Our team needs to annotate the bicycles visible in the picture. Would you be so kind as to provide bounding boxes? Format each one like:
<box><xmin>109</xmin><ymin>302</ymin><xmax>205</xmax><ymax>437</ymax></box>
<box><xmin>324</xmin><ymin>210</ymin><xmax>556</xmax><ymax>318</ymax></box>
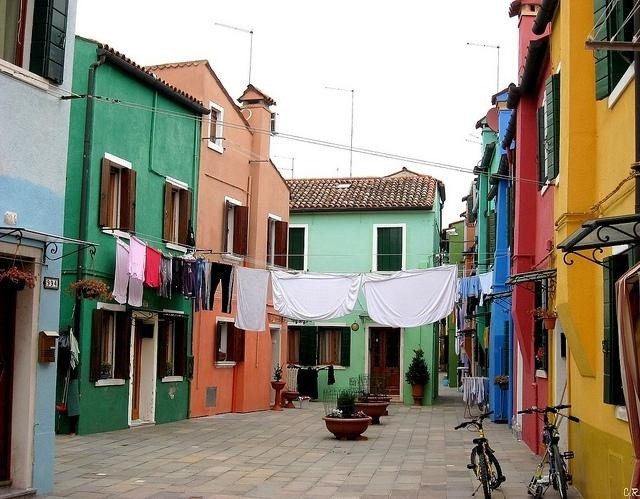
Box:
<box><xmin>454</xmin><ymin>410</ymin><xmax>506</xmax><ymax>499</ymax></box>
<box><xmin>517</xmin><ymin>403</ymin><xmax>579</xmax><ymax>498</ymax></box>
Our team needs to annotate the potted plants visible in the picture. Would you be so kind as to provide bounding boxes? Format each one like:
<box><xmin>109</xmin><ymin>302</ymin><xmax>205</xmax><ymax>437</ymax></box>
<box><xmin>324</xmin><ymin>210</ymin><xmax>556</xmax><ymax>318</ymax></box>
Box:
<box><xmin>493</xmin><ymin>373</ymin><xmax>509</xmax><ymax>391</ymax></box>
<box><xmin>271</xmin><ymin>361</ymin><xmax>286</xmax><ymax>410</ymax></box>
<box><xmin>98</xmin><ymin>360</ymin><xmax>113</xmax><ymax>379</ymax></box>
<box><xmin>322</xmin><ymin>374</ymin><xmax>392</xmax><ymax>440</ymax></box>
<box><xmin>283</xmin><ymin>390</ymin><xmax>299</xmax><ymax>408</ymax></box>
<box><xmin>404</xmin><ymin>347</ymin><xmax>430</xmax><ymax>406</ymax></box>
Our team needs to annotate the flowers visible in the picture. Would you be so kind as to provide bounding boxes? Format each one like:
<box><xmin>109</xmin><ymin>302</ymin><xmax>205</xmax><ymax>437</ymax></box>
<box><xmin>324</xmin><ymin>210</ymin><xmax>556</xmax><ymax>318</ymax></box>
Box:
<box><xmin>530</xmin><ymin>304</ymin><xmax>559</xmax><ymax>319</ymax></box>
<box><xmin>63</xmin><ymin>278</ymin><xmax>115</xmax><ymax>302</ymax></box>
<box><xmin>0</xmin><ymin>265</ymin><xmax>37</xmax><ymax>290</ymax></box>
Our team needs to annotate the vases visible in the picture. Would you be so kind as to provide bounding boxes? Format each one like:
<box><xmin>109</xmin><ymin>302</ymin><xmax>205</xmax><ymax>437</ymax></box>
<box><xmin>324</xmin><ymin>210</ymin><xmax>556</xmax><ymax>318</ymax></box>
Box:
<box><xmin>543</xmin><ymin>318</ymin><xmax>557</xmax><ymax>329</ymax></box>
<box><xmin>4</xmin><ymin>277</ymin><xmax>25</xmax><ymax>292</ymax></box>
<box><xmin>78</xmin><ymin>288</ymin><xmax>97</xmax><ymax>300</ymax></box>
